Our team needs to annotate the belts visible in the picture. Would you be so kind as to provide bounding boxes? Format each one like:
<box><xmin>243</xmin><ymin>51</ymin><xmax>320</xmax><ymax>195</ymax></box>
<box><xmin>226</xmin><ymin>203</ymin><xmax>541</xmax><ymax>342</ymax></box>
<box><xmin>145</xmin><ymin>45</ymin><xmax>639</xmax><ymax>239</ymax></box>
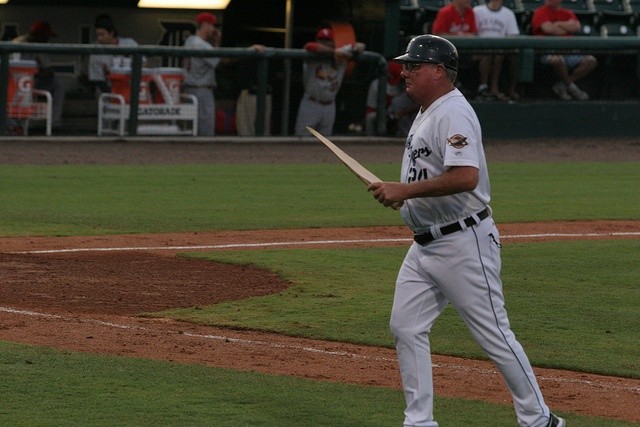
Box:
<box><xmin>308</xmin><ymin>96</ymin><xmax>333</xmax><ymax>105</ymax></box>
<box><xmin>415</xmin><ymin>207</ymin><xmax>488</xmax><ymax>243</ymax></box>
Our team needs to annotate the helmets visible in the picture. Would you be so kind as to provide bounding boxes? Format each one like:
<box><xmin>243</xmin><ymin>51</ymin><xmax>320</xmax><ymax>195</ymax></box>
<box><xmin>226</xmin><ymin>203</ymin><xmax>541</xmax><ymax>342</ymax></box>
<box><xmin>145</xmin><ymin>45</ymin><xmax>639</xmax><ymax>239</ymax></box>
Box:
<box><xmin>316</xmin><ymin>27</ymin><xmax>335</xmax><ymax>42</ymax></box>
<box><xmin>395</xmin><ymin>34</ymin><xmax>459</xmax><ymax>71</ymax></box>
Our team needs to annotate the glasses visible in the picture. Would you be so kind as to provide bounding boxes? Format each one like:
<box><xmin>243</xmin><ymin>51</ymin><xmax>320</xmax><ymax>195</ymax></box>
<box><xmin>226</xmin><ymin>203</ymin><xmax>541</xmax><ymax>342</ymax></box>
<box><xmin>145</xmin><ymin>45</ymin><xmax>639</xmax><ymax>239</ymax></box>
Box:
<box><xmin>404</xmin><ymin>62</ymin><xmax>433</xmax><ymax>68</ymax></box>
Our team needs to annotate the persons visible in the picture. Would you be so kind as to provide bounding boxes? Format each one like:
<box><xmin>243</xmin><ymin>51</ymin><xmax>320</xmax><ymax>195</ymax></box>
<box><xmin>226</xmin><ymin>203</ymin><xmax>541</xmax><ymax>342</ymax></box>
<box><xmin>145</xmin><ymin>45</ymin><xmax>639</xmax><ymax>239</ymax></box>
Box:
<box><xmin>293</xmin><ymin>28</ymin><xmax>365</xmax><ymax>136</ymax></box>
<box><xmin>473</xmin><ymin>0</ymin><xmax>521</xmax><ymax>101</ymax></box>
<box><xmin>8</xmin><ymin>18</ymin><xmax>54</xmax><ymax>91</ymax></box>
<box><xmin>364</xmin><ymin>60</ymin><xmax>419</xmax><ymax>136</ymax></box>
<box><xmin>531</xmin><ymin>0</ymin><xmax>597</xmax><ymax>101</ymax></box>
<box><xmin>431</xmin><ymin>0</ymin><xmax>496</xmax><ymax>101</ymax></box>
<box><xmin>87</xmin><ymin>16</ymin><xmax>148</xmax><ymax>93</ymax></box>
<box><xmin>366</xmin><ymin>33</ymin><xmax>567</xmax><ymax>427</ymax></box>
<box><xmin>179</xmin><ymin>12</ymin><xmax>267</xmax><ymax>136</ymax></box>
<box><xmin>236</xmin><ymin>42</ymin><xmax>273</xmax><ymax>139</ymax></box>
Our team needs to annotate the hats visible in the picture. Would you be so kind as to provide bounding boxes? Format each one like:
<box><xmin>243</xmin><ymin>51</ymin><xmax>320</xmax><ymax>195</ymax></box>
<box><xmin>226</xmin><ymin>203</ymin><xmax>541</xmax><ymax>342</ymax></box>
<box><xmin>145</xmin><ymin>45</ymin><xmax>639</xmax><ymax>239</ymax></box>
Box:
<box><xmin>30</xmin><ymin>20</ymin><xmax>58</xmax><ymax>38</ymax></box>
<box><xmin>94</xmin><ymin>16</ymin><xmax>120</xmax><ymax>34</ymax></box>
<box><xmin>195</xmin><ymin>12</ymin><xmax>218</xmax><ymax>27</ymax></box>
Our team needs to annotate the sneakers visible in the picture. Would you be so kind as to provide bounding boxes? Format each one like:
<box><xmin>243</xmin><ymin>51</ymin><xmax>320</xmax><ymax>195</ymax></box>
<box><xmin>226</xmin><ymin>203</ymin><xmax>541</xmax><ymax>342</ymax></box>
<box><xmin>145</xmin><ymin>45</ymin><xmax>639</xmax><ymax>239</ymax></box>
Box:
<box><xmin>545</xmin><ymin>410</ymin><xmax>565</xmax><ymax>427</ymax></box>
<box><xmin>553</xmin><ymin>81</ymin><xmax>572</xmax><ymax>100</ymax></box>
<box><xmin>567</xmin><ymin>83</ymin><xmax>589</xmax><ymax>101</ymax></box>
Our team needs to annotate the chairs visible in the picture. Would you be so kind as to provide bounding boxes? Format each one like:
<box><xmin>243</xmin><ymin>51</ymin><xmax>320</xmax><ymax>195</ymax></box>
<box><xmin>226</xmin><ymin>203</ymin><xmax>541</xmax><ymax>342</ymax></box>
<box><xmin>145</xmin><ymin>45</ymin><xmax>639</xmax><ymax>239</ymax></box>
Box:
<box><xmin>397</xmin><ymin>0</ymin><xmax>638</xmax><ymax>99</ymax></box>
<box><xmin>156</xmin><ymin>19</ymin><xmax>196</xmax><ymax>68</ymax></box>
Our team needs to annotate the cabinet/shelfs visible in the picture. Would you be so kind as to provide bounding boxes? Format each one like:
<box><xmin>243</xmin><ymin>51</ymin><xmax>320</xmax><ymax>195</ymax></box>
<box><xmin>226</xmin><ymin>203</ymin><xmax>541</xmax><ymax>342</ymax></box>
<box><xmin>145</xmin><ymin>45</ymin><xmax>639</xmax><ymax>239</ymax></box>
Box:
<box><xmin>6</xmin><ymin>88</ymin><xmax>56</xmax><ymax>137</ymax></box>
<box><xmin>96</xmin><ymin>93</ymin><xmax>200</xmax><ymax>137</ymax></box>
